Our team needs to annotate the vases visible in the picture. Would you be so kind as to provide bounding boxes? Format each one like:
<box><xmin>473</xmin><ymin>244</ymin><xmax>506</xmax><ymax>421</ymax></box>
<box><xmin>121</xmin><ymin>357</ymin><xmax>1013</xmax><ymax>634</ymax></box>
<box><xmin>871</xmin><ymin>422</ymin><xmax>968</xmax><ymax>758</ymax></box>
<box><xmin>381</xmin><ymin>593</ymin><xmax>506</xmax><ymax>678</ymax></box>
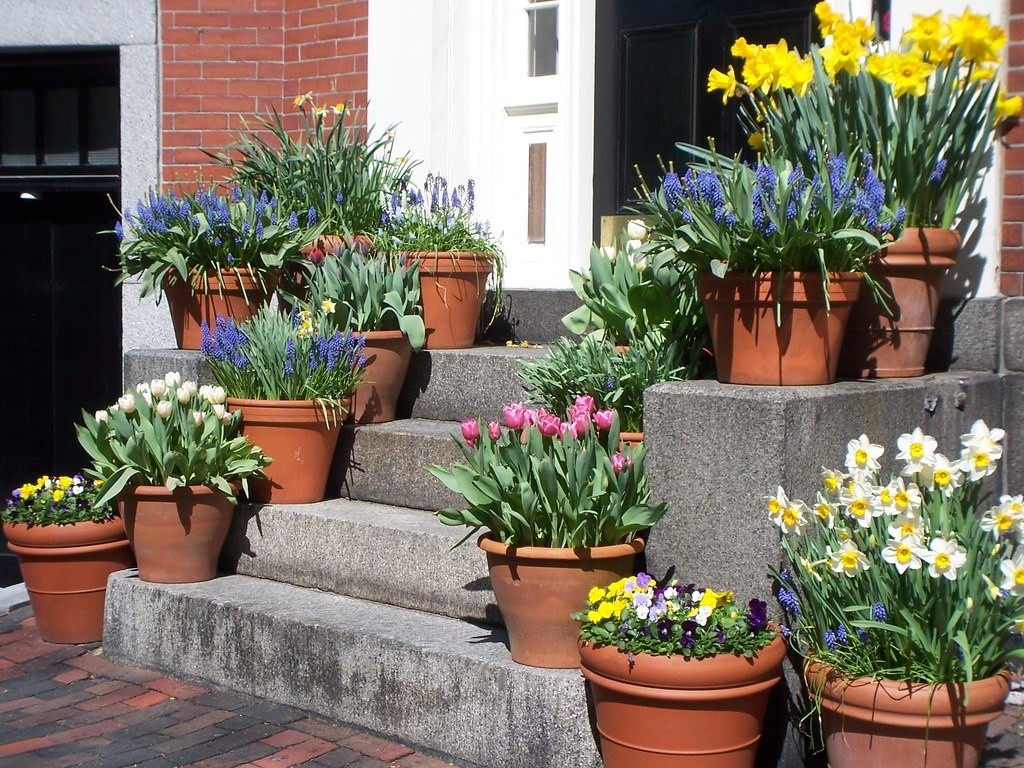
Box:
<box><xmin>285</xmin><ymin>235</ymin><xmax>372</xmax><ymax>310</ymax></box>
<box><xmin>160</xmin><ymin>268</ymin><xmax>283</xmax><ymax>351</ymax></box>
<box><xmin>837</xmin><ymin>226</ymin><xmax>963</xmax><ymax>378</ymax></box>
<box><xmin>576</xmin><ymin>621</ymin><xmax>790</xmax><ymax>768</ymax></box>
<box><xmin>596</xmin><ymin>432</ymin><xmax>643</xmax><ymax>454</ymax></box>
<box><xmin>383</xmin><ymin>250</ymin><xmax>493</xmax><ymax>349</ymax></box>
<box><xmin>3</xmin><ymin>515</ymin><xmax>136</xmax><ymax>644</ymax></box>
<box><xmin>801</xmin><ymin>648</ymin><xmax>1012</xmax><ymax>767</ymax></box>
<box><xmin>338</xmin><ymin>329</ymin><xmax>412</xmax><ymax>424</ymax></box>
<box><xmin>477</xmin><ymin>531</ymin><xmax>646</xmax><ymax>668</ymax></box>
<box><xmin>696</xmin><ymin>271</ymin><xmax>864</xmax><ymax>386</ymax></box>
<box><xmin>224</xmin><ymin>397</ymin><xmax>352</xmax><ymax>504</ymax></box>
<box><xmin>614</xmin><ymin>347</ymin><xmax>712</xmax><ymax>378</ymax></box>
<box><xmin>114</xmin><ymin>481</ymin><xmax>245</xmax><ymax>584</ymax></box>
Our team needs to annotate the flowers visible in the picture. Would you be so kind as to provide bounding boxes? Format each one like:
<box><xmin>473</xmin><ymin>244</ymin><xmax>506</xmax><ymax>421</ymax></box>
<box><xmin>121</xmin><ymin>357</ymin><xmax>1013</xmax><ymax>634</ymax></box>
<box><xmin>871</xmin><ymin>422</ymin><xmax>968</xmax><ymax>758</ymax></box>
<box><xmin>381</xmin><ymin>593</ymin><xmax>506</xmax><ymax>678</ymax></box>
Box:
<box><xmin>515</xmin><ymin>0</ymin><xmax>1024</xmax><ymax>432</ymax></box>
<box><xmin>569</xmin><ymin>565</ymin><xmax>786</xmax><ymax>668</ymax></box>
<box><xmin>73</xmin><ymin>371</ymin><xmax>275</xmax><ymax>506</ymax></box>
<box><xmin>761</xmin><ymin>417</ymin><xmax>1024</xmax><ymax>768</ymax></box>
<box><xmin>92</xmin><ymin>90</ymin><xmax>509</xmax><ymax>431</ymax></box>
<box><xmin>0</xmin><ymin>473</ymin><xmax>119</xmax><ymax>527</ymax></box>
<box><xmin>421</xmin><ymin>393</ymin><xmax>670</xmax><ymax>554</ymax></box>
